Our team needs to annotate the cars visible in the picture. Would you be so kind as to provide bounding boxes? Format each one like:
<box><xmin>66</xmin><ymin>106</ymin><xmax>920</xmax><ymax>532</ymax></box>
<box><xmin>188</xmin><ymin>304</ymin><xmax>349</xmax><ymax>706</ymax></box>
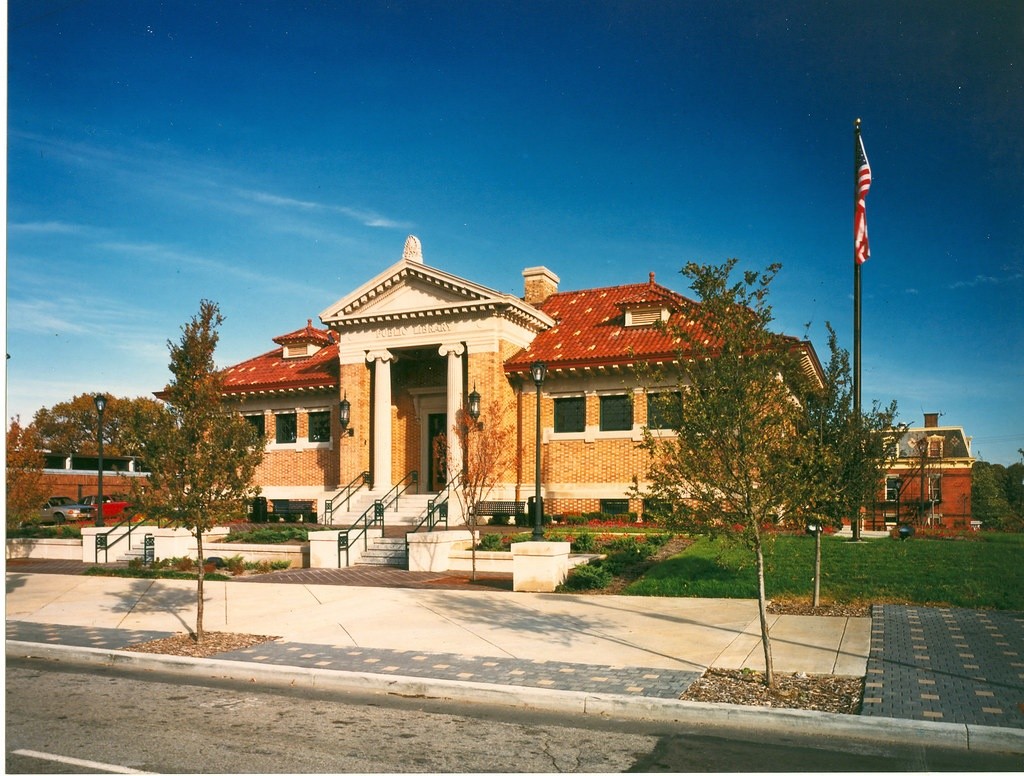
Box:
<box><xmin>78</xmin><ymin>495</ymin><xmax>133</xmax><ymax>519</ymax></box>
<box><xmin>29</xmin><ymin>494</ymin><xmax>96</xmax><ymax>524</ymax></box>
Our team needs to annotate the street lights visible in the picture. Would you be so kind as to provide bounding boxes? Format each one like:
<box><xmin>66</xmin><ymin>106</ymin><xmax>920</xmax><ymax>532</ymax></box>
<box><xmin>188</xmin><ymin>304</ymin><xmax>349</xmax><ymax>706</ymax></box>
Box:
<box><xmin>92</xmin><ymin>393</ymin><xmax>108</xmax><ymax>528</ymax></box>
<box><xmin>530</xmin><ymin>357</ymin><xmax>548</xmax><ymax>542</ymax></box>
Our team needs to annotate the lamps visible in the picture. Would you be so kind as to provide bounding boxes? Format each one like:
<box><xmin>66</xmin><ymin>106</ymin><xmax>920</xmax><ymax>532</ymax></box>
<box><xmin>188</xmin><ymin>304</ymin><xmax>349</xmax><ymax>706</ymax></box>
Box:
<box><xmin>468</xmin><ymin>379</ymin><xmax>483</xmax><ymax>431</ymax></box>
<box><xmin>339</xmin><ymin>390</ymin><xmax>354</xmax><ymax>436</ymax></box>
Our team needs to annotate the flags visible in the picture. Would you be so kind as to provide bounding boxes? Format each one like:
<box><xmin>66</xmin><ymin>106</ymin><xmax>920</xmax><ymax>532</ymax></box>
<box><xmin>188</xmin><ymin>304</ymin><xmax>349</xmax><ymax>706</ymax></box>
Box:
<box><xmin>854</xmin><ymin>128</ymin><xmax>872</xmax><ymax>265</ymax></box>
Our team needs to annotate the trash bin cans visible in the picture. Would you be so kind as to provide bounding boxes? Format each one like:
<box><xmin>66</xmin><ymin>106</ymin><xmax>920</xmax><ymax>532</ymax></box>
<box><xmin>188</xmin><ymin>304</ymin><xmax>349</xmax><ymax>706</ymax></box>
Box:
<box><xmin>252</xmin><ymin>496</ymin><xmax>268</xmax><ymax>523</ymax></box>
<box><xmin>527</xmin><ymin>496</ymin><xmax>544</xmax><ymax>527</ymax></box>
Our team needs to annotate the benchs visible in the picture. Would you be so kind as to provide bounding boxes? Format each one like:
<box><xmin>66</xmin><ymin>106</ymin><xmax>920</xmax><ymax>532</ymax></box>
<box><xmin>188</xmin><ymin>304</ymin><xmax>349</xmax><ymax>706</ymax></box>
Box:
<box><xmin>468</xmin><ymin>501</ymin><xmax>526</xmax><ymax>525</ymax></box>
<box><xmin>269</xmin><ymin>499</ymin><xmax>315</xmax><ymax>524</ymax></box>
